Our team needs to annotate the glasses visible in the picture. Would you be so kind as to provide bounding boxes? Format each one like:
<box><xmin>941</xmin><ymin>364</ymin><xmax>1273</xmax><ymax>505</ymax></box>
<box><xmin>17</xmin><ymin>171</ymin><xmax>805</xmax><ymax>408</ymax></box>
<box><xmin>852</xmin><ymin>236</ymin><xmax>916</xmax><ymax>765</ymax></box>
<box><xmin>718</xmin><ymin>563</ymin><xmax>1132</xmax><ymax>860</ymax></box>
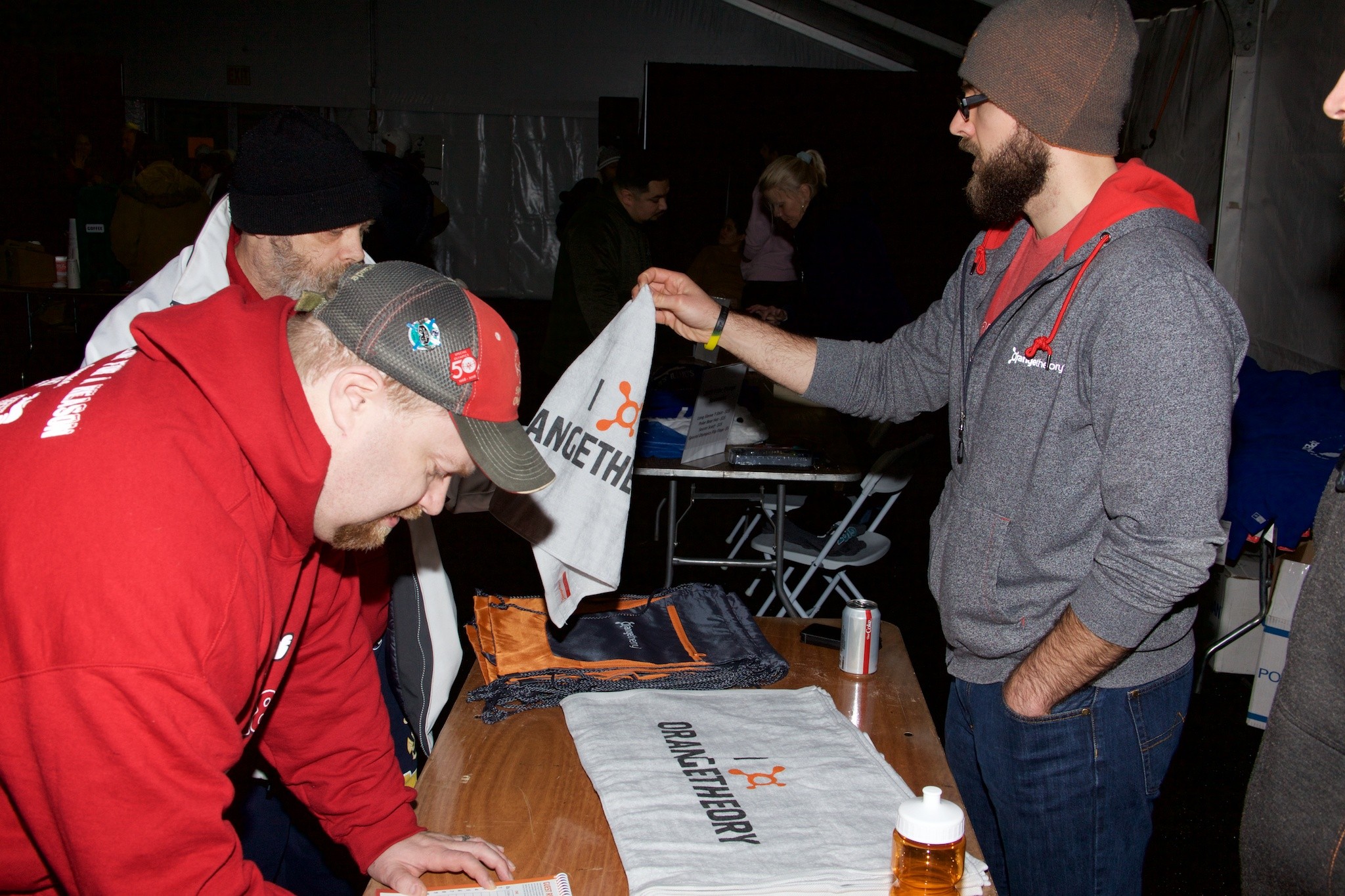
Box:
<box><xmin>956</xmin><ymin>94</ymin><xmax>989</xmax><ymax>122</ymax></box>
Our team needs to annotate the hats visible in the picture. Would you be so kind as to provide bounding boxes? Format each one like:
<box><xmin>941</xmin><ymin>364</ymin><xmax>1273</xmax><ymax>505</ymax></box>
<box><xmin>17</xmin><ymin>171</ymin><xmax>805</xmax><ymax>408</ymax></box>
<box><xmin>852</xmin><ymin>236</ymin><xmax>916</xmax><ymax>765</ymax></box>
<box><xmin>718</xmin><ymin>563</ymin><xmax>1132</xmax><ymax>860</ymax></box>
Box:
<box><xmin>957</xmin><ymin>0</ymin><xmax>1139</xmax><ymax>158</ymax></box>
<box><xmin>293</xmin><ymin>258</ymin><xmax>556</xmax><ymax>495</ymax></box>
<box><xmin>230</xmin><ymin>115</ymin><xmax>392</xmax><ymax>236</ymax></box>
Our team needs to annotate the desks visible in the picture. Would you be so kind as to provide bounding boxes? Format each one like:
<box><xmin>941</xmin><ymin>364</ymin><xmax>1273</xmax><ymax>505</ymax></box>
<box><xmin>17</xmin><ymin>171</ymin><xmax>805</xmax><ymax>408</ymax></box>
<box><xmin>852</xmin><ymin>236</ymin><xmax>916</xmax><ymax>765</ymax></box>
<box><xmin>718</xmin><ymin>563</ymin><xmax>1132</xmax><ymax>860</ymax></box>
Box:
<box><xmin>1192</xmin><ymin>357</ymin><xmax>1345</xmax><ymax>694</ymax></box>
<box><xmin>630</xmin><ymin>370</ymin><xmax>862</xmax><ymax>621</ymax></box>
<box><xmin>360</xmin><ymin>615</ymin><xmax>997</xmax><ymax>896</ymax></box>
<box><xmin>0</xmin><ymin>281</ymin><xmax>134</xmax><ymax>390</ymax></box>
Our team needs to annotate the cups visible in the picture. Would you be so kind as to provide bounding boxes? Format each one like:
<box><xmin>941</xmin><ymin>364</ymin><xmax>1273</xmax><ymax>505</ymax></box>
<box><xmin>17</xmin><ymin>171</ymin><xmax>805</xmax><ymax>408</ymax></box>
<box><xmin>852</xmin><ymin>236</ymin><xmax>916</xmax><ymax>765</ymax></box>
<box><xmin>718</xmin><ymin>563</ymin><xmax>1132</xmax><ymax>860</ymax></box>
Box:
<box><xmin>55</xmin><ymin>256</ymin><xmax>67</xmax><ymax>276</ymax></box>
<box><xmin>890</xmin><ymin>786</ymin><xmax>968</xmax><ymax>896</ymax></box>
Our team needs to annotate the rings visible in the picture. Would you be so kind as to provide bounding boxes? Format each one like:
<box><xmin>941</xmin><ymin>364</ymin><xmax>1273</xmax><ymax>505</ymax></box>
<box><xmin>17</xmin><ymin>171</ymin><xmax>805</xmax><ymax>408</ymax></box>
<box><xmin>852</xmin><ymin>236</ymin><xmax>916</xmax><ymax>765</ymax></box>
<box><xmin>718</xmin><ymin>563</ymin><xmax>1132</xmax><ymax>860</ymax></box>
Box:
<box><xmin>461</xmin><ymin>835</ymin><xmax>471</xmax><ymax>841</ymax></box>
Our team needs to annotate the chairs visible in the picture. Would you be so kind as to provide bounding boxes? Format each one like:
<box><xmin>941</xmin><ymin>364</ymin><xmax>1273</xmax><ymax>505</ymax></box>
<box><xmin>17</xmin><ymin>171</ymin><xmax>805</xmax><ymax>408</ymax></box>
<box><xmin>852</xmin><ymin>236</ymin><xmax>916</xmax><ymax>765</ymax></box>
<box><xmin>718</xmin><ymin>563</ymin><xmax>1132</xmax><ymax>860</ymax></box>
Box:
<box><xmin>721</xmin><ymin>490</ymin><xmax>806</xmax><ymax>598</ymax></box>
<box><xmin>746</xmin><ymin>430</ymin><xmax>937</xmax><ymax>620</ymax></box>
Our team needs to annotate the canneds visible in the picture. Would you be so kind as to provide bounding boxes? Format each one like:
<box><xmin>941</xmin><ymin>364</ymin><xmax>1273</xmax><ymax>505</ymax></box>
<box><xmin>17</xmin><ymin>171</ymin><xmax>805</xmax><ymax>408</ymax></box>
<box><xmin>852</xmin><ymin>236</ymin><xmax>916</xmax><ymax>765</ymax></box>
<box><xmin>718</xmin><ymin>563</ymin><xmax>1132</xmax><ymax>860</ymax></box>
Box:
<box><xmin>839</xmin><ymin>599</ymin><xmax>881</xmax><ymax>674</ymax></box>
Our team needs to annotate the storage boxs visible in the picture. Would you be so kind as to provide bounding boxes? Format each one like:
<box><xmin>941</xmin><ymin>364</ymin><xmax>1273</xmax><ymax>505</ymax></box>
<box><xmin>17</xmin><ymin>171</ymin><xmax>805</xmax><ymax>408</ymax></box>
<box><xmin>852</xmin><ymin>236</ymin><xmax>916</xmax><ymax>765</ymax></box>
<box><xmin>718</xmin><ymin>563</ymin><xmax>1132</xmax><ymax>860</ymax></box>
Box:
<box><xmin>1203</xmin><ymin>557</ymin><xmax>1276</xmax><ymax>677</ymax></box>
<box><xmin>0</xmin><ymin>240</ymin><xmax>58</xmax><ymax>289</ymax></box>
<box><xmin>1246</xmin><ymin>540</ymin><xmax>1316</xmax><ymax>731</ymax></box>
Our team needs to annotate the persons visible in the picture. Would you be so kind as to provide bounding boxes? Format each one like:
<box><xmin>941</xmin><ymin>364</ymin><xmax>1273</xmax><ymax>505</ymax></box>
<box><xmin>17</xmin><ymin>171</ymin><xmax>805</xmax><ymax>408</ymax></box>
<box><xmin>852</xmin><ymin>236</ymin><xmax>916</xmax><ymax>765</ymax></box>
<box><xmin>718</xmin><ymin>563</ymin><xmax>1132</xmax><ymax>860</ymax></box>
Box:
<box><xmin>9</xmin><ymin>110</ymin><xmax>480</xmax><ymax>345</ymax></box>
<box><xmin>689</xmin><ymin>215</ymin><xmax>751</xmax><ymax>314</ymax></box>
<box><xmin>739</xmin><ymin>124</ymin><xmax>805</xmax><ymax>336</ymax></box>
<box><xmin>579</xmin><ymin>146</ymin><xmax>626</xmax><ymax>217</ymax></box>
<box><xmin>1234</xmin><ymin>59</ymin><xmax>1345</xmax><ymax>896</ymax></box>
<box><xmin>626</xmin><ymin>0</ymin><xmax>1251</xmax><ymax>894</ymax></box>
<box><xmin>2</xmin><ymin>259</ymin><xmax>556</xmax><ymax>895</ymax></box>
<box><xmin>80</xmin><ymin>105</ymin><xmax>466</xmax><ymax>896</ymax></box>
<box><xmin>541</xmin><ymin>148</ymin><xmax>673</xmax><ymax>601</ymax></box>
<box><xmin>747</xmin><ymin>149</ymin><xmax>920</xmax><ymax>504</ymax></box>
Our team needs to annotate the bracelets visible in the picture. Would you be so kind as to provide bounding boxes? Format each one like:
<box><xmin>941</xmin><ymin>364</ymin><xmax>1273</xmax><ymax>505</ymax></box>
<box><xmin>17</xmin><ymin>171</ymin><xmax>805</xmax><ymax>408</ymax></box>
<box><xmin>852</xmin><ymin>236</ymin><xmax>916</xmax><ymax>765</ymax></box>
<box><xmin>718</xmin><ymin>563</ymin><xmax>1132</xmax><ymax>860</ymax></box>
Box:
<box><xmin>705</xmin><ymin>305</ymin><xmax>730</xmax><ymax>350</ymax></box>
